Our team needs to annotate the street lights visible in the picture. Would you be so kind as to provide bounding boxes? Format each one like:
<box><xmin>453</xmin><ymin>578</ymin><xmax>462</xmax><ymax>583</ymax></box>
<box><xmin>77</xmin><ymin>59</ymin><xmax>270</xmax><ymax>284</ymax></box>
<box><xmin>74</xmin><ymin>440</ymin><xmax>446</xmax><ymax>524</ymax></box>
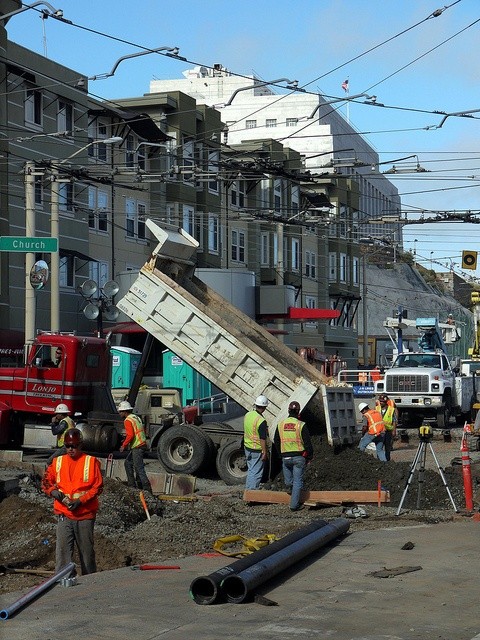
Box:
<box><xmin>47</xmin><ymin>136</ymin><xmax>124</xmax><ymax>333</ymax></box>
<box><xmin>276</xmin><ymin>207</ymin><xmax>329</xmax><ymax>344</ymax></box>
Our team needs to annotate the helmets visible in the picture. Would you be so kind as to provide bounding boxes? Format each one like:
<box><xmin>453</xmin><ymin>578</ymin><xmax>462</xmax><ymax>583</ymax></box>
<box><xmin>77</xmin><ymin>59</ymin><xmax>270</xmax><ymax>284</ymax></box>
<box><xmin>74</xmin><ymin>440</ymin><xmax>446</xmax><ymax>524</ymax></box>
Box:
<box><xmin>254</xmin><ymin>395</ymin><xmax>268</xmax><ymax>407</ymax></box>
<box><xmin>117</xmin><ymin>401</ymin><xmax>133</xmax><ymax>411</ymax></box>
<box><xmin>289</xmin><ymin>401</ymin><xmax>300</xmax><ymax>410</ymax></box>
<box><xmin>379</xmin><ymin>394</ymin><xmax>388</xmax><ymax>401</ymax></box>
<box><xmin>55</xmin><ymin>403</ymin><xmax>70</xmax><ymax>413</ymax></box>
<box><xmin>358</xmin><ymin>403</ymin><xmax>368</xmax><ymax>412</ymax></box>
<box><xmin>64</xmin><ymin>428</ymin><xmax>82</xmax><ymax>442</ymax></box>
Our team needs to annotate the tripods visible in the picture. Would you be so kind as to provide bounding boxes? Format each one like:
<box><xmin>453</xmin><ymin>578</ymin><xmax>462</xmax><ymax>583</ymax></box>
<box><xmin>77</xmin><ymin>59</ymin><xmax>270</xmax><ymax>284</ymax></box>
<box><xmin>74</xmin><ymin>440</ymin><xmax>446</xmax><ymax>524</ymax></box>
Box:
<box><xmin>397</xmin><ymin>437</ymin><xmax>461</xmax><ymax>516</ymax></box>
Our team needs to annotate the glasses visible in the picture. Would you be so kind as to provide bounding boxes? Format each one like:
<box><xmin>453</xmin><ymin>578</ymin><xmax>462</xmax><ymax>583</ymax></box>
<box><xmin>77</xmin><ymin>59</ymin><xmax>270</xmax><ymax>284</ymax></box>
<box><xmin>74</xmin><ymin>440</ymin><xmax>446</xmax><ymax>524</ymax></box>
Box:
<box><xmin>65</xmin><ymin>443</ymin><xmax>78</xmax><ymax>449</ymax></box>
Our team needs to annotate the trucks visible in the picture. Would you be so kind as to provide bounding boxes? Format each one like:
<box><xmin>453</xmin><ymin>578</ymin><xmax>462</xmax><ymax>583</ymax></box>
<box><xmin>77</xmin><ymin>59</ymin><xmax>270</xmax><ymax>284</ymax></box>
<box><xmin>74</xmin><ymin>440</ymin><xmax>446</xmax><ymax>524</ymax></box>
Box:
<box><xmin>0</xmin><ymin>218</ymin><xmax>360</xmax><ymax>487</ymax></box>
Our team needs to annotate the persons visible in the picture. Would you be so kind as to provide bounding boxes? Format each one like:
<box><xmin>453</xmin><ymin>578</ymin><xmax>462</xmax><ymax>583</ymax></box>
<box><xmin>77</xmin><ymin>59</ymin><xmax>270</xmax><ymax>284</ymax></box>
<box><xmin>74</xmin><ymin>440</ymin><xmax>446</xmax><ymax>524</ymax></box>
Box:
<box><xmin>375</xmin><ymin>399</ymin><xmax>399</xmax><ymax>420</ymax></box>
<box><xmin>274</xmin><ymin>401</ymin><xmax>313</xmax><ymax>511</ymax></box>
<box><xmin>40</xmin><ymin>428</ymin><xmax>103</xmax><ymax>575</ymax></box>
<box><xmin>118</xmin><ymin>401</ymin><xmax>152</xmax><ymax>494</ymax></box>
<box><xmin>358</xmin><ymin>403</ymin><xmax>387</xmax><ymax>461</ymax></box>
<box><xmin>55</xmin><ymin>348</ymin><xmax>62</xmax><ymax>368</ymax></box>
<box><xmin>243</xmin><ymin>395</ymin><xmax>269</xmax><ymax>490</ymax></box>
<box><xmin>375</xmin><ymin>394</ymin><xmax>396</xmax><ymax>461</ymax></box>
<box><xmin>46</xmin><ymin>404</ymin><xmax>77</xmax><ymax>466</ymax></box>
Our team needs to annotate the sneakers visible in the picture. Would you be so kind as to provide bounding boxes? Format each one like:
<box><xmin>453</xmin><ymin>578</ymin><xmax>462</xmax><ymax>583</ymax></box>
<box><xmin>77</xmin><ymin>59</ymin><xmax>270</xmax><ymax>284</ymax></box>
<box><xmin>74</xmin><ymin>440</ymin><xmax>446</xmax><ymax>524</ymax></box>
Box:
<box><xmin>292</xmin><ymin>503</ymin><xmax>304</xmax><ymax>510</ymax></box>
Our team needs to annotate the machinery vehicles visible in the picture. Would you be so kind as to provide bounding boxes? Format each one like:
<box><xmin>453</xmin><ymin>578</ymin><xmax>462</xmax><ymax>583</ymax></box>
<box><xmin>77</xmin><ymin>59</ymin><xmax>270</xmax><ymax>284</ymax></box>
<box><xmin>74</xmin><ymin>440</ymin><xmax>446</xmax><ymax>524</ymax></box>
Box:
<box><xmin>374</xmin><ymin>317</ymin><xmax>480</xmax><ymax>428</ymax></box>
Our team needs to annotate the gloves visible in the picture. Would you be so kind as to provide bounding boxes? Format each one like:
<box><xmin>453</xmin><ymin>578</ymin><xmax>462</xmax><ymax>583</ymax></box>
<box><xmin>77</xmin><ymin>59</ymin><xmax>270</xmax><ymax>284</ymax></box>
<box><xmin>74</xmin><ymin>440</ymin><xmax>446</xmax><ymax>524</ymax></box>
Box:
<box><xmin>68</xmin><ymin>499</ymin><xmax>80</xmax><ymax>510</ymax></box>
<box><xmin>120</xmin><ymin>446</ymin><xmax>124</xmax><ymax>452</ymax></box>
<box><xmin>51</xmin><ymin>490</ymin><xmax>64</xmax><ymax>501</ymax></box>
<box><xmin>52</xmin><ymin>417</ymin><xmax>57</xmax><ymax>422</ymax></box>
